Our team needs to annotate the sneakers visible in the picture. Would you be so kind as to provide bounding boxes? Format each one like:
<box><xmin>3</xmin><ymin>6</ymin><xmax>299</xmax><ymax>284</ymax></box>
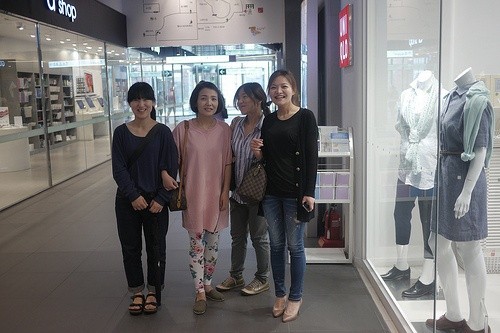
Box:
<box><xmin>240</xmin><ymin>277</ymin><xmax>270</xmax><ymax>295</ymax></box>
<box><xmin>215</xmin><ymin>276</ymin><xmax>246</xmax><ymax>292</ymax></box>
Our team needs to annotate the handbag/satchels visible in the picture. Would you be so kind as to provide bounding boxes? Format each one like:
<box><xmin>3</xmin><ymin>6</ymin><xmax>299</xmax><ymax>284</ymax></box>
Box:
<box><xmin>237</xmin><ymin>152</ymin><xmax>270</xmax><ymax>206</ymax></box>
<box><xmin>167</xmin><ymin>119</ymin><xmax>189</xmax><ymax>212</ymax></box>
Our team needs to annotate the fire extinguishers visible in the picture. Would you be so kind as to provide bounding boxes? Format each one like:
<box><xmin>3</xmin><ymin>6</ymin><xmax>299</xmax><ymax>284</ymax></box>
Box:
<box><xmin>322</xmin><ymin>204</ymin><xmax>340</xmax><ymax>240</ymax></box>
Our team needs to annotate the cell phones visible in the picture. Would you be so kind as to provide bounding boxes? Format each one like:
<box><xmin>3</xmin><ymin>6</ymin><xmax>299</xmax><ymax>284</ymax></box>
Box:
<box><xmin>302</xmin><ymin>203</ymin><xmax>310</xmax><ymax>213</ymax></box>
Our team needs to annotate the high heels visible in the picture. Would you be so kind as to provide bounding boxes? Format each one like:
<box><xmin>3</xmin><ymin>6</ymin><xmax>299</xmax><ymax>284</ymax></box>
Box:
<box><xmin>271</xmin><ymin>295</ymin><xmax>287</xmax><ymax>317</ymax></box>
<box><xmin>282</xmin><ymin>298</ymin><xmax>303</xmax><ymax>322</ymax></box>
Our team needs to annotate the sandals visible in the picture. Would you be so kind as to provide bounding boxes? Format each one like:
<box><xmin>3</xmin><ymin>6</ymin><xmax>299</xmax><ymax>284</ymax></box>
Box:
<box><xmin>128</xmin><ymin>294</ymin><xmax>146</xmax><ymax>315</ymax></box>
<box><xmin>142</xmin><ymin>293</ymin><xmax>158</xmax><ymax>314</ymax></box>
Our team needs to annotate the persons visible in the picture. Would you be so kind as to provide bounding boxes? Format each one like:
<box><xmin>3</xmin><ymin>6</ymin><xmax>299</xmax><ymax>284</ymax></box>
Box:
<box><xmin>161</xmin><ymin>80</ymin><xmax>232</xmax><ymax>314</ymax></box>
<box><xmin>426</xmin><ymin>68</ymin><xmax>495</xmax><ymax>333</ymax></box>
<box><xmin>157</xmin><ymin>86</ymin><xmax>179</xmax><ymax>123</ymax></box>
<box><xmin>216</xmin><ymin>96</ymin><xmax>226</xmax><ymax>122</ymax></box>
<box><xmin>250</xmin><ymin>70</ymin><xmax>318</xmax><ymax>323</ymax></box>
<box><xmin>381</xmin><ymin>71</ymin><xmax>449</xmax><ymax>298</ymax></box>
<box><xmin>215</xmin><ymin>82</ymin><xmax>272</xmax><ymax>295</ymax></box>
<box><xmin>112</xmin><ymin>82</ymin><xmax>179</xmax><ymax>313</ymax></box>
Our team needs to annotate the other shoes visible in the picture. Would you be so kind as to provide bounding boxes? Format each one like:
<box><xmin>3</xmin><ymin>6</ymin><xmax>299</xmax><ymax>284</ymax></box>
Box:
<box><xmin>426</xmin><ymin>313</ymin><xmax>466</xmax><ymax>330</ymax></box>
<box><xmin>192</xmin><ymin>297</ymin><xmax>207</xmax><ymax>315</ymax></box>
<box><xmin>455</xmin><ymin>321</ymin><xmax>491</xmax><ymax>333</ymax></box>
<box><xmin>206</xmin><ymin>288</ymin><xmax>225</xmax><ymax>302</ymax></box>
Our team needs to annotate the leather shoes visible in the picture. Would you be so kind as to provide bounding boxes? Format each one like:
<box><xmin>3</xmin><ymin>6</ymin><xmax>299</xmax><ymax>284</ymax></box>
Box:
<box><xmin>380</xmin><ymin>266</ymin><xmax>411</xmax><ymax>281</ymax></box>
<box><xmin>402</xmin><ymin>279</ymin><xmax>435</xmax><ymax>298</ymax></box>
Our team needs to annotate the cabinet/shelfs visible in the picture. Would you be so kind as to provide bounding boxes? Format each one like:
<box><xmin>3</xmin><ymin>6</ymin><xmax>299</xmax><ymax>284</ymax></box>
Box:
<box><xmin>289</xmin><ymin>126</ymin><xmax>354</xmax><ymax>263</ymax></box>
<box><xmin>17</xmin><ymin>71</ymin><xmax>79</xmax><ymax>156</ymax></box>
